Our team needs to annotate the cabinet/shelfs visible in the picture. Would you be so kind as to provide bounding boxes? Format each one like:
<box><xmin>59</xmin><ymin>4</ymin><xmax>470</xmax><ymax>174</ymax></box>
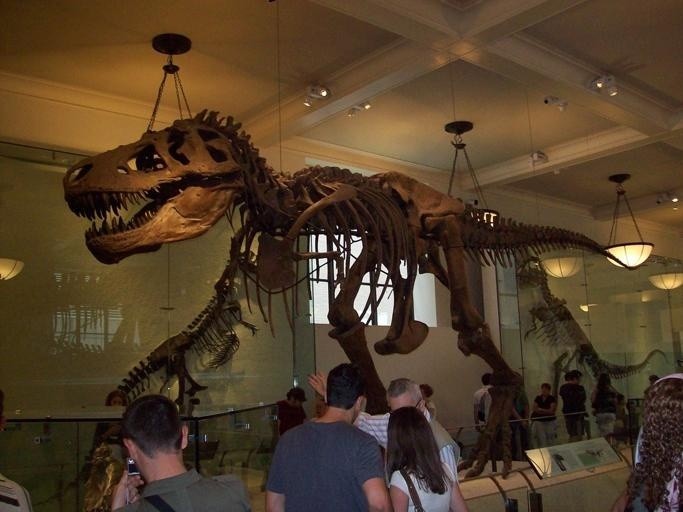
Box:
<box><xmin>0</xmin><ymin>400</ymin><xmax>286</xmax><ymax>512</ymax></box>
<box><xmin>457</xmin><ymin>441</ymin><xmax>638</xmax><ymax>512</ymax></box>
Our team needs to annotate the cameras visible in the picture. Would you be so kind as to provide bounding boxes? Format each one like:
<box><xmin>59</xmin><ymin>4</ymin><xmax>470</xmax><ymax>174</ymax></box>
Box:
<box><xmin>126</xmin><ymin>457</ymin><xmax>139</xmax><ymax>475</ymax></box>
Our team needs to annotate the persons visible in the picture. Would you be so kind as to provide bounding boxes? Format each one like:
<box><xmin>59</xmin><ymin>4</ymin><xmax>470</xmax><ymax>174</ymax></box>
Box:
<box><xmin>473</xmin><ymin>376</ymin><xmax>530</xmax><ymax>461</ymax></box>
<box><xmin>264</xmin><ymin>362</ymin><xmax>394</xmax><ymax>512</ymax></box>
<box><xmin>610</xmin><ymin>373</ymin><xmax>683</xmax><ymax>512</ymax></box>
<box><xmin>309</xmin><ymin>372</ymin><xmax>461</xmax><ymax>476</ymax></box>
<box><xmin>420</xmin><ymin>384</ymin><xmax>436</xmax><ymax>418</ymax></box>
<box><xmin>386</xmin><ymin>406</ymin><xmax>470</xmax><ymax>512</ymax></box>
<box><xmin>533</xmin><ymin>372</ymin><xmax>623</xmax><ymax>447</ymax></box>
<box><xmin>91</xmin><ymin>389</ymin><xmax>128</xmax><ymax>454</ymax></box>
<box><xmin>274</xmin><ymin>387</ymin><xmax>308</xmax><ymax>443</ymax></box>
<box><xmin>104</xmin><ymin>395</ymin><xmax>252</xmax><ymax>511</ymax></box>
<box><xmin>1</xmin><ymin>390</ymin><xmax>33</xmax><ymax>511</ymax></box>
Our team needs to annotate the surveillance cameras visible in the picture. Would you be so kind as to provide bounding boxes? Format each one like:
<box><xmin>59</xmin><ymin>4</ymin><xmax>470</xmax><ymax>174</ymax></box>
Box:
<box><xmin>543</xmin><ymin>95</ymin><xmax>568</xmax><ymax>107</ymax></box>
<box><xmin>656</xmin><ymin>191</ymin><xmax>669</xmax><ymax>204</ymax></box>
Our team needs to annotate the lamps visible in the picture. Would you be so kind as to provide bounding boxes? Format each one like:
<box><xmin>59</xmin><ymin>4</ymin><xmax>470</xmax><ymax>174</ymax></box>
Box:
<box><xmin>586</xmin><ymin>70</ymin><xmax>621</xmax><ymax>99</ymax></box>
<box><xmin>540</xmin><ymin>252</ymin><xmax>586</xmax><ymax>282</ymax></box>
<box><xmin>601</xmin><ymin>172</ymin><xmax>656</xmax><ymax>271</ymax></box>
<box><xmin>665</xmin><ymin>191</ymin><xmax>682</xmax><ymax>214</ymax></box>
<box><xmin>542</xmin><ymin>92</ymin><xmax>570</xmax><ymax>115</ymax></box>
<box><xmin>645</xmin><ymin>262</ymin><xmax>682</xmax><ymax>292</ymax></box>
<box><xmin>441</xmin><ymin>117</ymin><xmax>501</xmax><ymax>230</ymax></box>
<box><xmin>343</xmin><ymin>101</ymin><xmax>373</xmax><ymax>122</ymax></box>
<box><xmin>0</xmin><ymin>254</ymin><xmax>27</xmax><ymax>282</ymax></box>
<box><xmin>298</xmin><ymin>81</ymin><xmax>333</xmax><ymax>111</ymax></box>
